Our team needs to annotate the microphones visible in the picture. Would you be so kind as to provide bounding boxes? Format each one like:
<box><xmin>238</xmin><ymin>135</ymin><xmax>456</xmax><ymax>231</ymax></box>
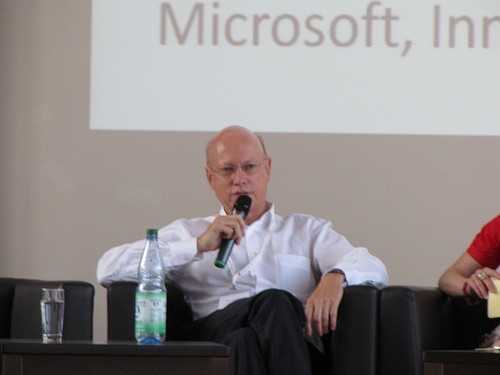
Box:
<box><xmin>215</xmin><ymin>194</ymin><xmax>252</xmax><ymax>268</ymax></box>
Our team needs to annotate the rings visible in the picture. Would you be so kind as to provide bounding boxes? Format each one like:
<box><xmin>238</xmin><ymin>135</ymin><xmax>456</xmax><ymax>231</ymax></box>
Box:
<box><xmin>478</xmin><ymin>271</ymin><xmax>489</xmax><ymax>281</ymax></box>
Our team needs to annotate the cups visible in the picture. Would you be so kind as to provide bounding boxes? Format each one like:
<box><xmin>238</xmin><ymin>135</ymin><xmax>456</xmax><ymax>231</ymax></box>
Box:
<box><xmin>40</xmin><ymin>288</ymin><xmax>64</xmax><ymax>343</ymax></box>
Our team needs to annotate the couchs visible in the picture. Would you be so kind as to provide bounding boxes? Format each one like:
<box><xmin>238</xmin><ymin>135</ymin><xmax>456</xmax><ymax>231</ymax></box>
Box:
<box><xmin>107</xmin><ymin>282</ymin><xmax>500</xmax><ymax>375</ymax></box>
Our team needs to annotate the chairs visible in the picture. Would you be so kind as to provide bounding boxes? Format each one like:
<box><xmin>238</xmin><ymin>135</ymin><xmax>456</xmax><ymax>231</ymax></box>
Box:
<box><xmin>0</xmin><ymin>277</ymin><xmax>95</xmax><ymax>344</ymax></box>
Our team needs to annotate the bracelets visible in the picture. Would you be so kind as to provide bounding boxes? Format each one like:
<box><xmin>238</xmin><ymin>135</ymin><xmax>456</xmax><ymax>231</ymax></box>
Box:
<box><xmin>463</xmin><ymin>281</ymin><xmax>471</xmax><ymax>298</ymax></box>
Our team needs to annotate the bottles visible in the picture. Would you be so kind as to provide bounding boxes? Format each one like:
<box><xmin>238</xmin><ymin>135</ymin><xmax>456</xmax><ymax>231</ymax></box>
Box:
<box><xmin>134</xmin><ymin>228</ymin><xmax>167</xmax><ymax>344</ymax></box>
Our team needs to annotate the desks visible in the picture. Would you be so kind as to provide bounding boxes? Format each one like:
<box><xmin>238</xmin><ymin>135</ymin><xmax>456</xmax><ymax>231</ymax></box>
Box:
<box><xmin>422</xmin><ymin>350</ymin><xmax>500</xmax><ymax>375</ymax></box>
<box><xmin>0</xmin><ymin>340</ymin><xmax>229</xmax><ymax>375</ymax></box>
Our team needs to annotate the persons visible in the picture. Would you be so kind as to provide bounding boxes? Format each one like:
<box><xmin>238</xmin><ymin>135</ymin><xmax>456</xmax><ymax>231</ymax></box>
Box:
<box><xmin>97</xmin><ymin>125</ymin><xmax>388</xmax><ymax>375</ymax></box>
<box><xmin>438</xmin><ymin>214</ymin><xmax>500</xmax><ymax>300</ymax></box>
